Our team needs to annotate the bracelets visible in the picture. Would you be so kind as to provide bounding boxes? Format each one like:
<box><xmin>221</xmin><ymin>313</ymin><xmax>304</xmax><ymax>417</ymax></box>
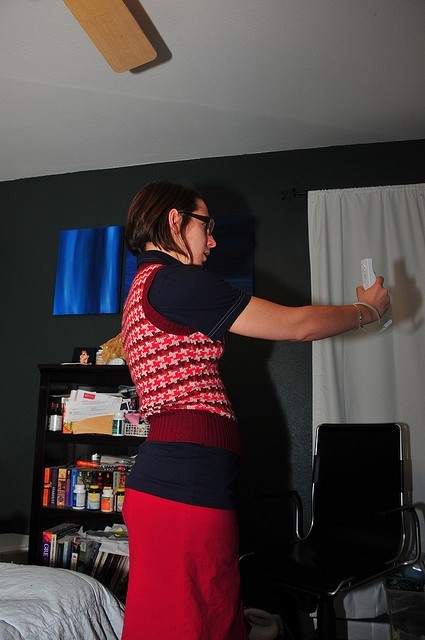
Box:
<box><xmin>352</xmin><ymin>304</ymin><xmax>364</xmax><ymax>332</ymax></box>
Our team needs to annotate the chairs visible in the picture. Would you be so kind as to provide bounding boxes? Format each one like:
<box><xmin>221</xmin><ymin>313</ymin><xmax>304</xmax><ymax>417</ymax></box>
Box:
<box><xmin>239</xmin><ymin>422</ymin><xmax>424</xmax><ymax>639</ymax></box>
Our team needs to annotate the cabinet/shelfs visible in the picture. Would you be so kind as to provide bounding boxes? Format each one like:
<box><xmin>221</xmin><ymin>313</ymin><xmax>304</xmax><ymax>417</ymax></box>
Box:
<box><xmin>29</xmin><ymin>362</ymin><xmax>150</xmax><ymax>601</ymax></box>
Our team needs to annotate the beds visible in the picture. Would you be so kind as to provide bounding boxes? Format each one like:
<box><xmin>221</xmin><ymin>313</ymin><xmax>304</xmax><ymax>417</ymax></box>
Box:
<box><xmin>0</xmin><ymin>562</ymin><xmax>125</xmax><ymax>640</ymax></box>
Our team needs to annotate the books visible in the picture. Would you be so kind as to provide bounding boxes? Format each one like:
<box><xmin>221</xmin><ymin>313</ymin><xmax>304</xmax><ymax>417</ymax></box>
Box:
<box><xmin>40</xmin><ymin>465</ymin><xmax>132</xmax><ymax>518</ymax></box>
<box><xmin>35</xmin><ymin>522</ymin><xmax>130</xmax><ymax>605</ymax></box>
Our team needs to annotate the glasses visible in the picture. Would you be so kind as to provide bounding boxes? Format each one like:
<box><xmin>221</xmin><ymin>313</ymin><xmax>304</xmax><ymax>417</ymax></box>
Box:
<box><xmin>179</xmin><ymin>209</ymin><xmax>217</xmax><ymax>236</ymax></box>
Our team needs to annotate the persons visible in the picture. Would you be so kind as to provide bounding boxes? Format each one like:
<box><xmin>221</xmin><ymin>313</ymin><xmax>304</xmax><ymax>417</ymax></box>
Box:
<box><xmin>120</xmin><ymin>179</ymin><xmax>391</xmax><ymax>639</ymax></box>
